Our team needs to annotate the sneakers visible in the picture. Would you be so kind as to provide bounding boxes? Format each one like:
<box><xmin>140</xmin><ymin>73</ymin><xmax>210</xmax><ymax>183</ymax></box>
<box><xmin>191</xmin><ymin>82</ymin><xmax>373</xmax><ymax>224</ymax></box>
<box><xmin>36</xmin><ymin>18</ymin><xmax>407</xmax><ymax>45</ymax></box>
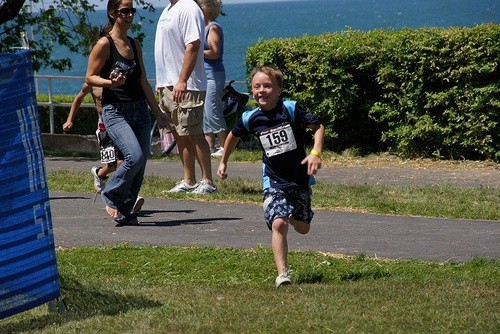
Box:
<box><xmin>130</xmin><ymin>196</ymin><xmax>144</xmax><ymax>213</ymax></box>
<box><xmin>91</xmin><ymin>166</ymin><xmax>109</xmax><ymax>193</ymax></box>
<box><xmin>210</xmin><ymin>146</ymin><xmax>224</xmax><ymax>157</ymax></box>
<box><xmin>186</xmin><ymin>181</ymin><xmax>217</xmax><ymax>194</ymax></box>
<box><xmin>163</xmin><ymin>180</ymin><xmax>200</xmax><ymax>194</ymax></box>
<box><xmin>276</xmin><ymin>267</ymin><xmax>292</xmax><ymax>288</ymax></box>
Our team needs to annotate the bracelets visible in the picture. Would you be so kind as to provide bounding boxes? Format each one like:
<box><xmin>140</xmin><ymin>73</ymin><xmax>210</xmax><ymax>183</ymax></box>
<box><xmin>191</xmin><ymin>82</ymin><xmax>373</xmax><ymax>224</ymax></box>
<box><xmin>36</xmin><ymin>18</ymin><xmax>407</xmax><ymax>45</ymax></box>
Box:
<box><xmin>311</xmin><ymin>151</ymin><xmax>322</xmax><ymax>158</ymax></box>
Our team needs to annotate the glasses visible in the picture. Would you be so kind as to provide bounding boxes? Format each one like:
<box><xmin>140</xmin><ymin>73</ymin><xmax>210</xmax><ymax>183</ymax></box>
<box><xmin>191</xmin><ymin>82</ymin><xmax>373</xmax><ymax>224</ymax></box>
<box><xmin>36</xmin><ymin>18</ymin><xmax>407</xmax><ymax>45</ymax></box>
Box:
<box><xmin>115</xmin><ymin>8</ymin><xmax>136</xmax><ymax>16</ymax></box>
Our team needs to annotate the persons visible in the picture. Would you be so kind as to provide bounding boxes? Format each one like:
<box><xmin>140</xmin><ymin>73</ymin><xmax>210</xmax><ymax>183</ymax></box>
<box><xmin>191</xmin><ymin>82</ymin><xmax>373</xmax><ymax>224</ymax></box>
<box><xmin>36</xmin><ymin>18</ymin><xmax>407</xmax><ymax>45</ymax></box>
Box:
<box><xmin>155</xmin><ymin>1</ymin><xmax>217</xmax><ymax>194</ymax></box>
<box><xmin>86</xmin><ymin>0</ymin><xmax>174</xmax><ymax>222</ymax></box>
<box><xmin>63</xmin><ymin>38</ymin><xmax>123</xmax><ymax>193</ymax></box>
<box><xmin>199</xmin><ymin>0</ymin><xmax>227</xmax><ymax>157</ymax></box>
<box><xmin>217</xmin><ymin>67</ymin><xmax>325</xmax><ymax>288</ymax></box>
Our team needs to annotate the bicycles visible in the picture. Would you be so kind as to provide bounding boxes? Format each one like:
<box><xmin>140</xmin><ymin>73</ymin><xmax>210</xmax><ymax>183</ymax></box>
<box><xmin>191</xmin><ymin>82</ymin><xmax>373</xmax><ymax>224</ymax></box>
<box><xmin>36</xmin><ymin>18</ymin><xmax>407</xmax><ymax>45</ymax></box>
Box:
<box><xmin>148</xmin><ymin>117</ymin><xmax>178</xmax><ymax>158</ymax></box>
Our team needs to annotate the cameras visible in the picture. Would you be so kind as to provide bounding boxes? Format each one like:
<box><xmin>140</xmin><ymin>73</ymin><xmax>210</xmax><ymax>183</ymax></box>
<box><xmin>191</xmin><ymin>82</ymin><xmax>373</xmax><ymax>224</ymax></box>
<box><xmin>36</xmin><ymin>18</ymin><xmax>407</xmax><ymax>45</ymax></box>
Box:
<box><xmin>111</xmin><ymin>67</ymin><xmax>123</xmax><ymax>78</ymax></box>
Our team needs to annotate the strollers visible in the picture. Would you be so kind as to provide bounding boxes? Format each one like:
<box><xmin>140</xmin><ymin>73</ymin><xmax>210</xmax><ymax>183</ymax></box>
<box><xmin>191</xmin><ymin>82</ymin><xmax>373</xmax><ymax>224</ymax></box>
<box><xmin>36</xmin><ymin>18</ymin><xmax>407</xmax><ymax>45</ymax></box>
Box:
<box><xmin>222</xmin><ymin>80</ymin><xmax>261</xmax><ymax>150</ymax></box>
